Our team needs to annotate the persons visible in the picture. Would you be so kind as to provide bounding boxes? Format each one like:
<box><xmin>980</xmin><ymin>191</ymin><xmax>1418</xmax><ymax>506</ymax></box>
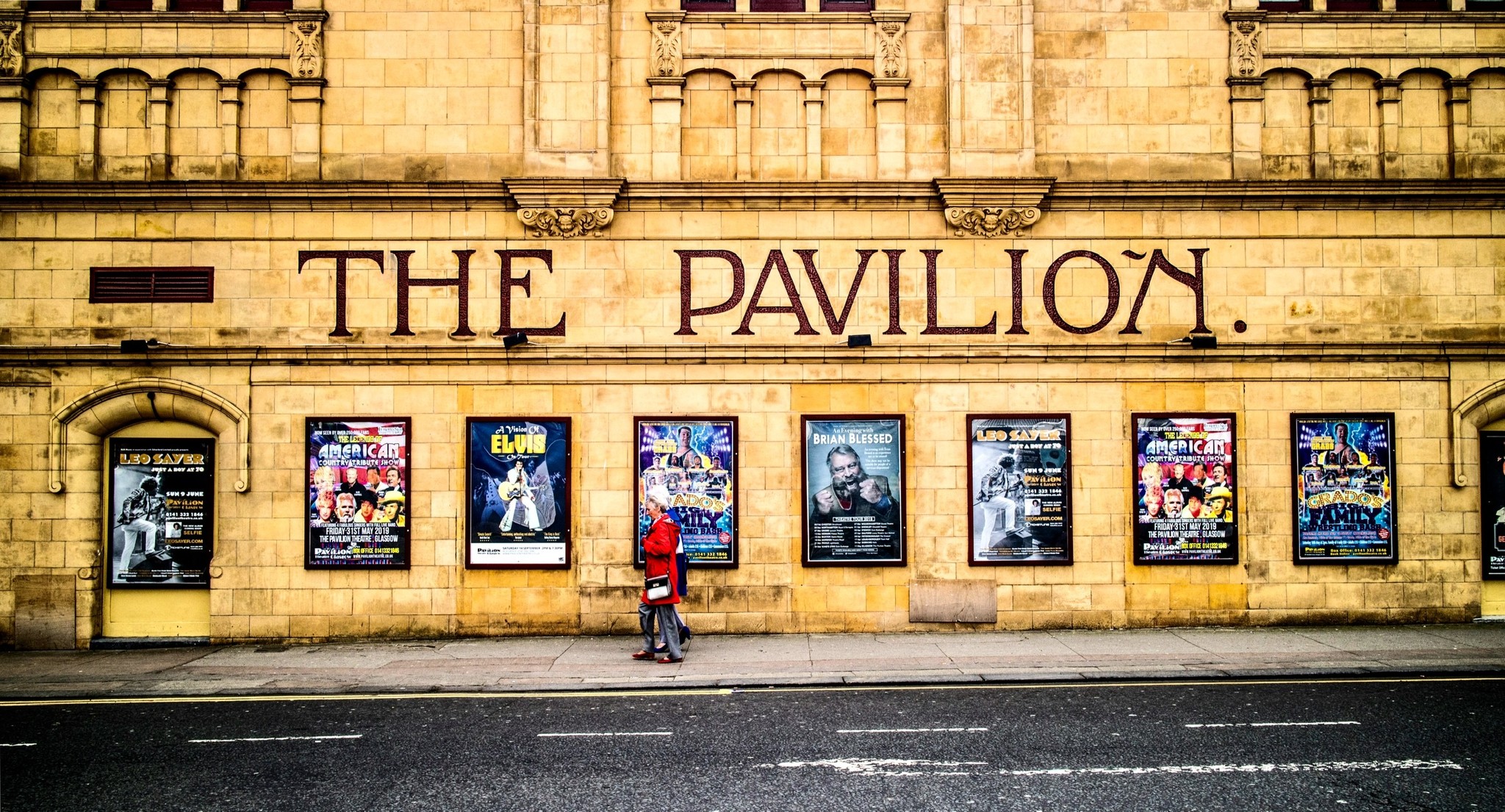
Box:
<box><xmin>641</xmin><ymin>427</ymin><xmax>732</xmax><ymax>499</ymax></box>
<box><xmin>170</xmin><ymin>523</ymin><xmax>181</xmax><ymax>538</ymax></box>
<box><xmin>809</xmin><ymin>444</ymin><xmax>899</xmax><ymax>561</ymax></box>
<box><xmin>1302</xmin><ymin>423</ymin><xmax>1390</xmax><ymax>496</ymax></box>
<box><xmin>633</xmin><ymin>485</ymin><xmax>691</xmax><ymax>664</ymax></box>
<box><xmin>310</xmin><ymin>466</ymin><xmax>405</xmax><ymax>527</ymax></box>
<box><xmin>117</xmin><ymin>476</ymin><xmax>166</xmax><ymax>578</ymax></box>
<box><xmin>1138</xmin><ymin>460</ymin><xmax>1233</xmax><ymax>523</ymax></box>
<box><xmin>976</xmin><ymin>454</ymin><xmax>1025</xmax><ymax>556</ymax></box>
<box><xmin>1030</xmin><ymin>500</ymin><xmax>1040</xmax><ymax>515</ymax></box>
<box><xmin>499</xmin><ymin>458</ymin><xmax>543</xmax><ymax>532</ymax></box>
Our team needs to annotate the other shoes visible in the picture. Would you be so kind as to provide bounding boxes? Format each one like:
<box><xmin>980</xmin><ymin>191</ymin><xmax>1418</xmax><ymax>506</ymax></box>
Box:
<box><xmin>1006</xmin><ymin>527</ymin><xmax>1025</xmax><ymax>536</ymax></box>
<box><xmin>631</xmin><ymin>652</ymin><xmax>655</xmax><ymax>660</ymax></box>
<box><xmin>979</xmin><ymin>553</ymin><xmax>986</xmax><ymax>557</ymax></box>
<box><xmin>145</xmin><ymin>550</ymin><xmax>165</xmax><ymax>559</ymax></box>
<box><xmin>118</xmin><ymin>574</ymin><xmax>126</xmax><ymax>580</ymax></box>
<box><xmin>658</xmin><ymin>657</ymin><xmax>683</xmax><ymax>663</ymax></box>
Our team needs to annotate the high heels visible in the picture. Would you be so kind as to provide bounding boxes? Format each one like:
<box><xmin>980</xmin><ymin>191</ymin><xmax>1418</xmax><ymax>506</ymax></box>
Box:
<box><xmin>679</xmin><ymin>626</ymin><xmax>690</xmax><ymax>645</ymax></box>
<box><xmin>530</xmin><ymin>528</ymin><xmax>544</xmax><ymax>531</ymax></box>
<box><xmin>654</xmin><ymin>643</ymin><xmax>670</xmax><ymax>653</ymax></box>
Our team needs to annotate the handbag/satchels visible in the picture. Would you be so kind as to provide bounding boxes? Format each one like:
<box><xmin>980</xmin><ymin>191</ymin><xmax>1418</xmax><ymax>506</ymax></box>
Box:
<box><xmin>645</xmin><ymin>574</ymin><xmax>672</xmax><ymax>600</ymax></box>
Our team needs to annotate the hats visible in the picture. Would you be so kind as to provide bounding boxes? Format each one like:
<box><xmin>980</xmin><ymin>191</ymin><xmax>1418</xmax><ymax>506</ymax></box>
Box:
<box><xmin>1203</xmin><ymin>486</ymin><xmax>1232</xmax><ymax>499</ymax></box>
<box><xmin>376</xmin><ymin>491</ymin><xmax>405</xmax><ymax>507</ymax></box>
